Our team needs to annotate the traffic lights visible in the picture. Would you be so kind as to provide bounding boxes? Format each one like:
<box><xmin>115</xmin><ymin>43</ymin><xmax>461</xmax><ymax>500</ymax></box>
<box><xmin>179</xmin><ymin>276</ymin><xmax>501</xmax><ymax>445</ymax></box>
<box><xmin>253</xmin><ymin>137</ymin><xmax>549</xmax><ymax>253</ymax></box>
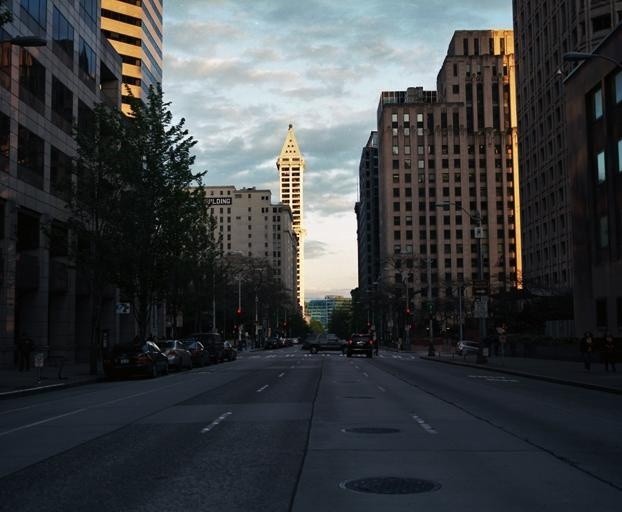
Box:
<box><xmin>427</xmin><ymin>301</ymin><xmax>435</xmax><ymax>315</ymax></box>
<box><xmin>236</xmin><ymin>309</ymin><xmax>241</xmax><ymax>318</ymax></box>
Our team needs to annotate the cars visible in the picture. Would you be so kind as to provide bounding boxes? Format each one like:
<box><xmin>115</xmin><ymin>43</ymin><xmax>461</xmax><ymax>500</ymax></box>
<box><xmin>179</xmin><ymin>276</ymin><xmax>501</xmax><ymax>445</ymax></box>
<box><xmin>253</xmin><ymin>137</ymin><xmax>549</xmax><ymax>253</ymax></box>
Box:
<box><xmin>302</xmin><ymin>332</ymin><xmax>348</xmax><ymax>355</ymax></box>
<box><xmin>98</xmin><ymin>330</ymin><xmax>301</xmax><ymax>379</ymax></box>
<box><xmin>456</xmin><ymin>339</ymin><xmax>482</xmax><ymax>355</ymax></box>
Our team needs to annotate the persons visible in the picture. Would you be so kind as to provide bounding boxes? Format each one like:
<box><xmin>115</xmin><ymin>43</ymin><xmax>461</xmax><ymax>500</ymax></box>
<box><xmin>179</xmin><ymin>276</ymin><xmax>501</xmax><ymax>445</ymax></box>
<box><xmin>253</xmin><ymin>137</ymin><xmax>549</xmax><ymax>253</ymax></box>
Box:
<box><xmin>600</xmin><ymin>330</ymin><xmax>617</xmax><ymax>373</ymax></box>
<box><xmin>578</xmin><ymin>328</ymin><xmax>596</xmax><ymax>375</ymax></box>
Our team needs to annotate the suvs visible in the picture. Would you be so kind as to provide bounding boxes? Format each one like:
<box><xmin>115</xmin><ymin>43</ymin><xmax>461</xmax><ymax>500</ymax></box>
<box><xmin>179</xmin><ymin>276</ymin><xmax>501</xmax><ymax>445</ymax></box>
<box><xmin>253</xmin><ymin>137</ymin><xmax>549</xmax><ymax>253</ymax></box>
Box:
<box><xmin>346</xmin><ymin>332</ymin><xmax>381</xmax><ymax>359</ymax></box>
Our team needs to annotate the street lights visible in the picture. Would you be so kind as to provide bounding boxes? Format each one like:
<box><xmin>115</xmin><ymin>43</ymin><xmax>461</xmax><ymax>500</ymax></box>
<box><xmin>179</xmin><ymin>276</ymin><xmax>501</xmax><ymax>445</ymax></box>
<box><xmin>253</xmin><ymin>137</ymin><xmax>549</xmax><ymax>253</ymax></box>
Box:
<box><xmin>253</xmin><ymin>295</ymin><xmax>260</xmax><ymax>343</ymax></box>
<box><xmin>354</xmin><ymin>265</ymin><xmax>411</xmax><ymax>347</ymax></box>
<box><xmin>561</xmin><ymin>50</ymin><xmax>622</xmax><ymax>72</ymax></box>
<box><xmin>456</xmin><ymin>271</ymin><xmax>465</xmax><ymax>342</ymax></box>
<box><xmin>429</xmin><ymin>200</ymin><xmax>488</xmax><ymax>335</ymax></box>
